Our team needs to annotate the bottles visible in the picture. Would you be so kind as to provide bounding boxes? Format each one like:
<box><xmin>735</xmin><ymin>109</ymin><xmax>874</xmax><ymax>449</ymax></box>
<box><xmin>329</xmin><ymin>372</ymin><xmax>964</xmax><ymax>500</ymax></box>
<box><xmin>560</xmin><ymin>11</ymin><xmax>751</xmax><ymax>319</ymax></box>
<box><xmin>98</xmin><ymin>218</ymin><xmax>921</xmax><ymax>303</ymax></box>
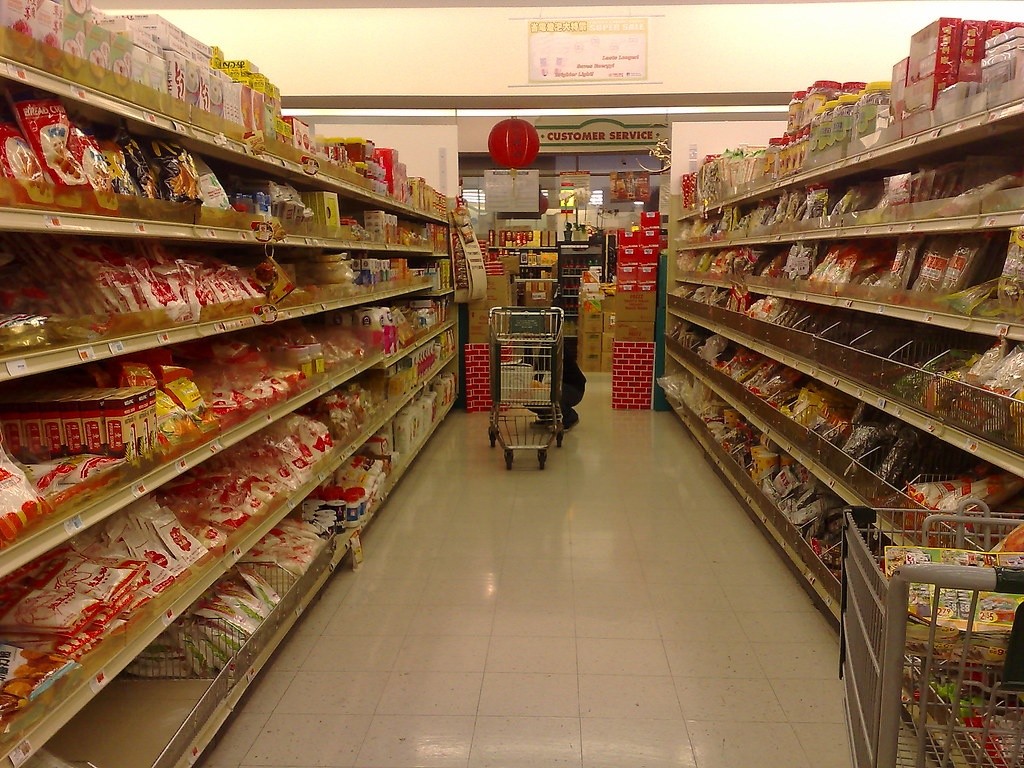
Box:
<box><xmin>289</xmin><ymin>342</ymin><xmax>325</xmax><ymax>387</ymax></box>
<box><xmin>563</xmin><ymin>256</ymin><xmax>602</xmax><ymax>337</ymax></box>
<box><xmin>763</xmin><ymin>79</ymin><xmax>891</xmax><ymax>186</ymax></box>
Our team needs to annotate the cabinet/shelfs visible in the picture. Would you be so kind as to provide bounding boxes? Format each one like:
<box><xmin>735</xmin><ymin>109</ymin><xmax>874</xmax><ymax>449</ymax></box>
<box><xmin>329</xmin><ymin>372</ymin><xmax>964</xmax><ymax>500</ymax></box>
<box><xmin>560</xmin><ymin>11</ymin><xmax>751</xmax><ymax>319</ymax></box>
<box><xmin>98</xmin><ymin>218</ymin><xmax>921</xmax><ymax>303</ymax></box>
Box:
<box><xmin>605</xmin><ymin>233</ymin><xmax>616</xmax><ymax>282</ymax></box>
<box><xmin>666</xmin><ymin>97</ymin><xmax>1024</xmax><ymax>767</ymax></box>
<box><xmin>486</xmin><ymin>245</ymin><xmax>559</xmax><ymax>307</ymax></box>
<box><xmin>0</xmin><ymin>20</ymin><xmax>460</xmax><ymax>768</ymax></box>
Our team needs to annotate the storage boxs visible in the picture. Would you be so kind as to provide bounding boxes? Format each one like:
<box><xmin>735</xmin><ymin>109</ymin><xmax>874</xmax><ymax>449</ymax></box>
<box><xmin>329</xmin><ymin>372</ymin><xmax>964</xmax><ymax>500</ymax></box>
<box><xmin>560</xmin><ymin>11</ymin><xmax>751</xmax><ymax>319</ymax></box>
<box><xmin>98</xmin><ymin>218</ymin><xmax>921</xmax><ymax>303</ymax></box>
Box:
<box><xmin>576</xmin><ymin>270</ymin><xmax>615</xmax><ymax>372</ymax></box>
<box><xmin>467</xmin><ymin>254</ymin><xmax>518</xmax><ymax>342</ymax></box>
<box><xmin>0</xmin><ymin>0</ymin><xmax>450</xmax><ymax>220</ymax></box>
<box><xmin>615</xmin><ymin>211</ymin><xmax>660</xmax><ymax>341</ymax></box>
<box><xmin>888</xmin><ymin>16</ymin><xmax>1024</xmax><ymax>124</ymax></box>
<box><xmin>525</xmin><ymin>279</ymin><xmax>552</xmax><ymax>306</ymax></box>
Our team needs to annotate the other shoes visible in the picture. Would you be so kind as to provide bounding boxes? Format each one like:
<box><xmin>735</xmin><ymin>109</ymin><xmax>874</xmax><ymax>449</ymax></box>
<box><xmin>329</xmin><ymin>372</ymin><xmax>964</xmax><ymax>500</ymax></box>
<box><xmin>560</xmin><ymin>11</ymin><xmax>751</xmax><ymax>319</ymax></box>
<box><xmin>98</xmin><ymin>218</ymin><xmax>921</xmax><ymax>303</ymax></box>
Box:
<box><xmin>531</xmin><ymin>420</ymin><xmax>550</xmax><ymax>428</ymax></box>
<box><xmin>550</xmin><ymin>419</ymin><xmax>580</xmax><ymax>435</ymax></box>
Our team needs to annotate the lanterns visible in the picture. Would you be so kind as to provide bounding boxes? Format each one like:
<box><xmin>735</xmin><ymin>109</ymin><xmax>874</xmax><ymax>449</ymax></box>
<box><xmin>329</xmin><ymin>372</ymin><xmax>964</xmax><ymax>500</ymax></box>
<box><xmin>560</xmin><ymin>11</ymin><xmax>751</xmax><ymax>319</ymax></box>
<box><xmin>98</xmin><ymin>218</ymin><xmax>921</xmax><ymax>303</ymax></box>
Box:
<box><xmin>538</xmin><ymin>192</ymin><xmax>548</xmax><ymax>215</ymax></box>
<box><xmin>488</xmin><ymin>116</ymin><xmax>539</xmax><ymax>199</ymax></box>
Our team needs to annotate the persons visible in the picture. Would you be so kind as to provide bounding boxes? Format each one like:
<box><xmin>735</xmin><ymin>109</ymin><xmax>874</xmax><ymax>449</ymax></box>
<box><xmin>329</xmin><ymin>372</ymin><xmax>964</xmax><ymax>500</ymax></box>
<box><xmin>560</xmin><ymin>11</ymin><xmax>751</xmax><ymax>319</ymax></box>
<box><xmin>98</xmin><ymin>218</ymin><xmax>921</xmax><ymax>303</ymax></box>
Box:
<box><xmin>519</xmin><ymin>318</ymin><xmax>587</xmax><ymax>432</ymax></box>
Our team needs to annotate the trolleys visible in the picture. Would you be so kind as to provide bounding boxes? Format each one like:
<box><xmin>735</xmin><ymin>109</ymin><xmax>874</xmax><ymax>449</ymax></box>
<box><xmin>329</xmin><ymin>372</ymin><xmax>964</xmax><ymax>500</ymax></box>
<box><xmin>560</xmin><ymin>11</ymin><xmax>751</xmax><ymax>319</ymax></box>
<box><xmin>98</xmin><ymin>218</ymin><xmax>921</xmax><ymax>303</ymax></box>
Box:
<box><xmin>488</xmin><ymin>306</ymin><xmax>564</xmax><ymax>471</ymax></box>
<box><xmin>838</xmin><ymin>498</ymin><xmax>1024</xmax><ymax>768</ymax></box>
